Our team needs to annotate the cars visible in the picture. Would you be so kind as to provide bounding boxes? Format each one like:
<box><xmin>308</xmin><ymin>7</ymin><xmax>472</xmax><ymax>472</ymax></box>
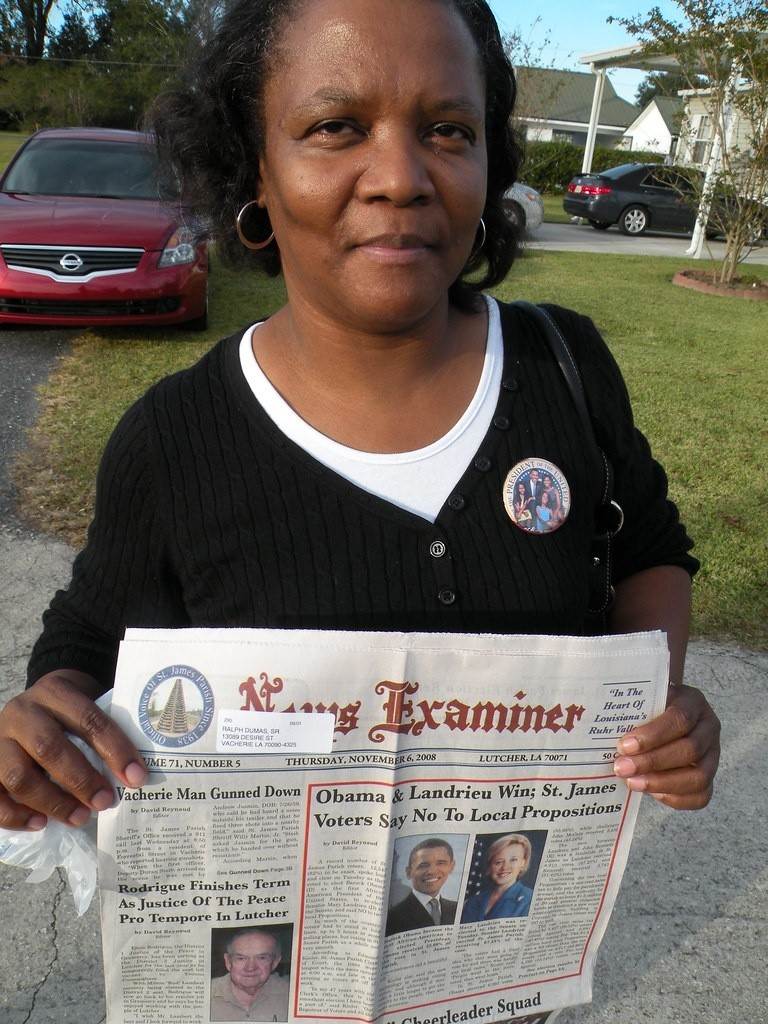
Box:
<box><xmin>501</xmin><ymin>183</ymin><xmax>544</xmax><ymax>233</ymax></box>
<box><xmin>563</xmin><ymin>160</ymin><xmax>768</xmax><ymax>246</ymax></box>
<box><xmin>0</xmin><ymin>125</ymin><xmax>216</xmax><ymax>335</ymax></box>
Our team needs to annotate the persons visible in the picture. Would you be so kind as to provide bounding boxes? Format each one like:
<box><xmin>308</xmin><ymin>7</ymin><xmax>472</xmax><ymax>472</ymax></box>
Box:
<box><xmin>384</xmin><ymin>834</ymin><xmax>536</xmax><ymax>937</ymax></box>
<box><xmin>211</xmin><ymin>931</ymin><xmax>290</xmax><ymax>1022</ymax></box>
<box><xmin>0</xmin><ymin>0</ymin><xmax>724</xmax><ymax>831</ymax></box>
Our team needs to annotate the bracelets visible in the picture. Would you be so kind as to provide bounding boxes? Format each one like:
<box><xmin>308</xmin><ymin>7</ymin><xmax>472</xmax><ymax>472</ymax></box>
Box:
<box><xmin>668</xmin><ymin>681</ymin><xmax>676</xmax><ymax>687</ymax></box>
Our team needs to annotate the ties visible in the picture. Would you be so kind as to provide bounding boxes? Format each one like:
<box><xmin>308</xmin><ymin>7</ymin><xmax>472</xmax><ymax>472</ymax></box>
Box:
<box><xmin>429</xmin><ymin>897</ymin><xmax>441</xmax><ymax>926</ymax></box>
